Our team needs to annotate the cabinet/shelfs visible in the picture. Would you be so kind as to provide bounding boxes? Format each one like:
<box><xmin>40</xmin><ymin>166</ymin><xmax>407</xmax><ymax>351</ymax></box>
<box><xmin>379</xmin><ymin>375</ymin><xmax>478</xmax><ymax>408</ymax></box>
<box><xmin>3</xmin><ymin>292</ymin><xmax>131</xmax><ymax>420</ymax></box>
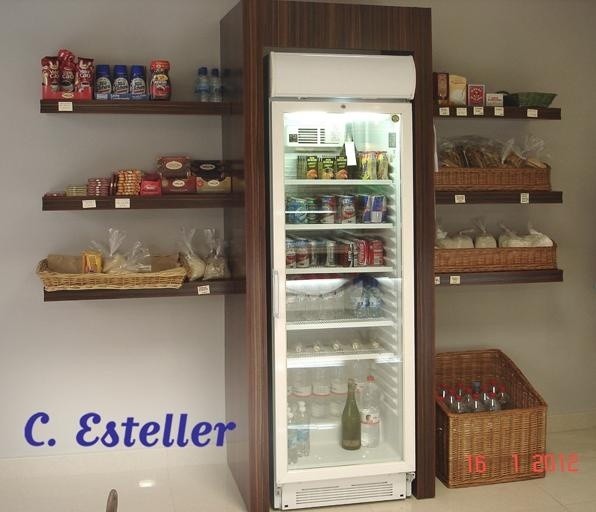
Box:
<box><xmin>40</xmin><ymin>99</ymin><xmax>246</xmax><ymax>302</ymax></box>
<box><xmin>220</xmin><ymin>0</ymin><xmax>435</xmax><ymax>512</ymax></box>
<box><xmin>435</xmin><ymin>104</ymin><xmax>563</xmax><ymax>287</ymax></box>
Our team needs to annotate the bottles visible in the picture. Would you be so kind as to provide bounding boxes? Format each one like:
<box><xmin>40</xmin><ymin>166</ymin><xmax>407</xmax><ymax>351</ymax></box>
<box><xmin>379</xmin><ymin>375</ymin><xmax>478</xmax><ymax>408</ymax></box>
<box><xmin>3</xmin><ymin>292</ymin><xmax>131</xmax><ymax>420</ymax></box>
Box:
<box><xmin>194</xmin><ymin>67</ymin><xmax>209</xmax><ymax>102</ymax></box>
<box><xmin>375</xmin><ymin>151</ymin><xmax>389</xmax><ymax>179</ymax></box>
<box><xmin>355</xmin><ymin>151</ymin><xmax>362</xmax><ymax>178</ymax></box>
<box><xmin>148</xmin><ymin>60</ymin><xmax>171</xmax><ymax>100</ymax></box>
<box><xmin>295</xmin><ymin>238</ymin><xmax>309</xmax><ymax>268</ymax></box>
<box><xmin>444</xmin><ymin>389</ymin><xmax>458</xmax><ymax>408</ymax></box>
<box><xmin>209</xmin><ymin>68</ymin><xmax>222</xmax><ymax>102</ymax></box>
<box><xmin>488</xmin><ymin>379</ymin><xmax>499</xmax><ymax>394</ymax></box>
<box><xmin>293</xmin><ymin>402</ymin><xmax>310</xmax><ymax>458</ymax></box>
<box><xmin>335</xmin><ymin>194</ymin><xmax>345</xmax><ymax>223</ymax></box>
<box><xmin>338</xmin><ymin>240</ymin><xmax>349</xmax><ymax>265</ymax></box>
<box><xmin>356</xmin><ymin>239</ymin><xmax>369</xmax><ymax>266</ymax></box>
<box><xmin>437</xmin><ymin>384</ymin><xmax>448</xmax><ymax>401</ymax></box>
<box><xmin>363</xmin><ymin>276</ymin><xmax>373</xmax><ymax>292</ymax></box>
<box><xmin>324</xmin><ymin>240</ymin><xmax>337</xmax><ymax>267</ymax></box>
<box><xmin>93</xmin><ymin>64</ymin><xmax>112</xmax><ymax>93</ymax></box>
<box><xmin>484</xmin><ymin>393</ymin><xmax>500</xmax><ymax>412</ymax></box>
<box><xmin>360</xmin><ymin>376</ymin><xmax>380</xmax><ymax>448</ymax></box>
<box><xmin>304</xmin><ymin>196</ymin><xmax>319</xmax><ymax>223</ymax></box>
<box><xmin>343</xmin><ymin>242</ymin><xmax>357</xmax><ymax>267</ymax></box>
<box><xmin>310</xmin><ymin>370</ymin><xmax>330</xmax><ymax>420</ymax></box>
<box><xmin>455</xmin><ymin>384</ymin><xmax>465</xmax><ymax>397</ymax></box>
<box><xmin>294</xmin><ymin>199</ymin><xmax>308</xmax><ymax>223</ymax></box>
<box><xmin>286</xmin><ymin>370</ymin><xmax>292</xmax><ymax>412</ymax></box>
<box><xmin>348</xmin><ymin>360</ymin><xmax>366</xmax><ymax>414</ymax></box>
<box><xmin>351</xmin><ymin>279</ymin><xmax>367</xmax><ymax>318</ymax></box>
<box><xmin>341</xmin><ymin>379</ymin><xmax>361</xmax><ymax>450</ymax></box>
<box><xmin>286</xmin><ymin>408</ymin><xmax>298</xmax><ymax>466</ymax></box>
<box><xmin>340</xmin><ymin>195</ymin><xmax>356</xmax><ymax>223</ymax></box>
<box><xmin>317</xmin><ymin>242</ymin><xmax>326</xmax><ymax>267</ymax></box>
<box><xmin>497</xmin><ymin>385</ymin><xmax>511</xmax><ymax>411</ymax></box>
<box><xmin>330</xmin><ymin>367</ymin><xmax>348</xmax><ymax>418</ymax></box>
<box><xmin>449</xmin><ymin>397</ymin><xmax>467</xmax><ymax>413</ymax></box>
<box><xmin>307</xmin><ymin>240</ymin><xmax>320</xmax><ymax>267</ymax></box>
<box><xmin>367</xmin><ymin>278</ymin><xmax>383</xmax><ymax>318</ymax></box>
<box><xmin>477</xmin><ymin>384</ymin><xmax>490</xmax><ymax>400</ymax></box>
<box><xmin>111</xmin><ymin>65</ymin><xmax>129</xmax><ymax>94</ymax></box>
<box><xmin>287</xmin><ymin>197</ymin><xmax>295</xmax><ymax>223</ymax></box>
<box><xmin>292</xmin><ymin>370</ymin><xmax>312</xmax><ymax>419</ymax></box>
<box><xmin>467</xmin><ymin>393</ymin><xmax>485</xmax><ymax>413</ymax></box>
<box><xmin>319</xmin><ymin>195</ymin><xmax>336</xmax><ymax>223</ymax></box>
<box><xmin>361</xmin><ymin>151</ymin><xmax>377</xmax><ymax>180</ymax></box>
<box><xmin>129</xmin><ymin>64</ymin><xmax>146</xmax><ymax>94</ymax></box>
<box><xmin>284</xmin><ymin>239</ymin><xmax>296</xmax><ymax>268</ymax></box>
<box><xmin>461</xmin><ymin>389</ymin><xmax>473</xmax><ymax>408</ymax></box>
<box><xmin>369</xmin><ymin>240</ymin><xmax>385</xmax><ymax>266</ymax></box>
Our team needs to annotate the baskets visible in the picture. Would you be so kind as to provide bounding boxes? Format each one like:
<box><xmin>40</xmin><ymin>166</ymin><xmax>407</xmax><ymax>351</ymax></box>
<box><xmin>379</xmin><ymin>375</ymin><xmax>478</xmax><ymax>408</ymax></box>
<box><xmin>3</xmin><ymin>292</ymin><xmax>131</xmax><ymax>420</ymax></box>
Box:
<box><xmin>35</xmin><ymin>257</ymin><xmax>187</xmax><ymax>292</ymax></box>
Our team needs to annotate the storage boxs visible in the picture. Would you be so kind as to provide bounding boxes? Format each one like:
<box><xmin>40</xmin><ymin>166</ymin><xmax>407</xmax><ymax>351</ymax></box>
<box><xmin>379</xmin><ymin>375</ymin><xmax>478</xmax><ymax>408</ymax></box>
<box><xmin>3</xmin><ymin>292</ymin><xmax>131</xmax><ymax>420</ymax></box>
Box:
<box><xmin>435</xmin><ymin>349</ymin><xmax>548</xmax><ymax>489</ymax></box>
